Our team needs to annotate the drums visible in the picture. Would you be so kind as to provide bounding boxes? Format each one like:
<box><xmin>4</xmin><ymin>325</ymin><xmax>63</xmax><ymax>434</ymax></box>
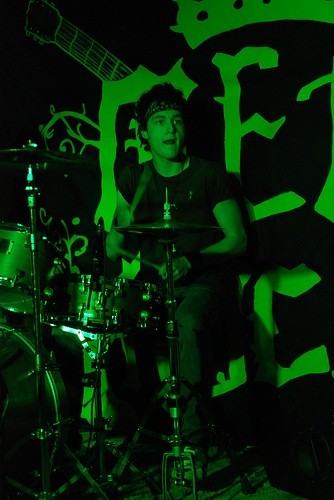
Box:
<box><xmin>40</xmin><ymin>271</ymin><xmax>167</xmax><ymax>343</ymax></box>
<box><xmin>0</xmin><ymin>223</ymin><xmax>47</xmax><ymax>292</ymax></box>
<box><xmin>0</xmin><ymin>323</ymin><xmax>85</xmax><ymax>500</ymax></box>
<box><xmin>0</xmin><ymin>288</ymin><xmax>45</xmax><ymax>332</ymax></box>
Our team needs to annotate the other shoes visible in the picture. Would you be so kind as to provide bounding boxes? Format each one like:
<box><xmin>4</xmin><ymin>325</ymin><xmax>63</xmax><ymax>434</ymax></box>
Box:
<box><xmin>171</xmin><ymin>444</ymin><xmax>208</xmax><ymax>483</ymax></box>
<box><xmin>83</xmin><ymin>435</ymin><xmax>138</xmax><ymax>471</ymax></box>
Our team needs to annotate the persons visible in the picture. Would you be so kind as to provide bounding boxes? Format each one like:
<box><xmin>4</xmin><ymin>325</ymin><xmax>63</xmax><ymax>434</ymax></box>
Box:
<box><xmin>106</xmin><ymin>82</ymin><xmax>248</xmax><ymax>483</ymax></box>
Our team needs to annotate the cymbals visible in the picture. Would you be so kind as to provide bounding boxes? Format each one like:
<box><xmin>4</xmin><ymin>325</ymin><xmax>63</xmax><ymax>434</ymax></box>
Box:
<box><xmin>112</xmin><ymin>219</ymin><xmax>226</xmax><ymax>240</ymax></box>
<box><xmin>0</xmin><ymin>146</ymin><xmax>100</xmax><ymax>171</ymax></box>
